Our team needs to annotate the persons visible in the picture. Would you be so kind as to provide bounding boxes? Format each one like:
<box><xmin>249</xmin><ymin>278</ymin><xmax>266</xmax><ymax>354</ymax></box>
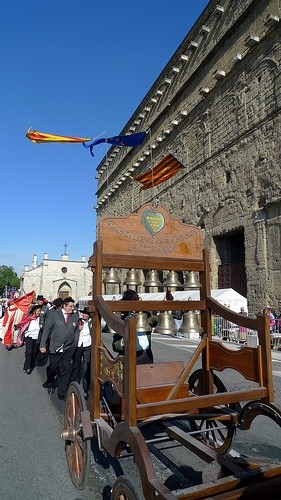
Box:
<box><xmin>264</xmin><ymin>306</ymin><xmax>281</xmax><ymax>351</ymax></box>
<box><xmin>20</xmin><ymin>295</ymin><xmax>93</xmax><ymax>400</ymax></box>
<box><xmin>3</xmin><ymin>301</ymin><xmax>24</xmax><ymax>350</ymax></box>
<box><xmin>113</xmin><ymin>290</ymin><xmax>154</xmax><ymax>365</ymax></box>
<box><xmin>239</xmin><ymin>307</ymin><xmax>247</xmax><ymax>332</ymax></box>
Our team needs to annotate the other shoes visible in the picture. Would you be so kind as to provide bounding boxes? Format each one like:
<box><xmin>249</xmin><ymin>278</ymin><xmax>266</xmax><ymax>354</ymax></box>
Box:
<box><xmin>59</xmin><ymin>397</ymin><xmax>66</xmax><ymax>401</ymax></box>
<box><xmin>26</xmin><ymin>368</ymin><xmax>31</xmax><ymax>374</ymax></box>
<box><xmin>6</xmin><ymin>345</ymin><xmax>13</xmax><ymax>350</ymax></box>
<box><xmin>47</xmin><ymin>387</ymin><xmax>55</xmax><ymax>395</ymax></box>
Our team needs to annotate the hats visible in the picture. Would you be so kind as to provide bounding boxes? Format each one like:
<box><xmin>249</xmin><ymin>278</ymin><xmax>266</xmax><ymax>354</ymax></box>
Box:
<box><xmin>36</xmin><ymin>295</ymin><xmax>45</xmax><ymax>300</ymax></box>
<box><xmin>29</xmin><ymin>305</ymin><xmax>41</xmax><ymax>314</ymax></box>
<box><xmin>35</xmin><ymin>353</ymin><xmax>48</xmax><ymax>367</ymax></box>
<box><xmin>78</xmin><ymin>307</ymin><xmax>88</xmax><ymax>314</ymax></box>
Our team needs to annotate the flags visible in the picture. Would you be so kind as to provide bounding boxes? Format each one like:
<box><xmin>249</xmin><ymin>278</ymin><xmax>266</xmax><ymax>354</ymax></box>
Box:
<box><xmin>132</xmin><ymin>154</ymin><xmax>182</xmax><ymax>190</ymax></box>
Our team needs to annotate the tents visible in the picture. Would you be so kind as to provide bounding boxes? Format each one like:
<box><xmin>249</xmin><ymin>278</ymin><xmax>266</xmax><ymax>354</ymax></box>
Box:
<box><xmin>78</xmin><ymin>288</ymin><xmax>248</xmax><ymax>313</ymax></box>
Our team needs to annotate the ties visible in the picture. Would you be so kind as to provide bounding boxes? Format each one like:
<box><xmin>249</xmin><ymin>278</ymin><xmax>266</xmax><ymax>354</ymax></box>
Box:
<box><xmin>66</xmin><ymin>313</ymin><xmax>69</xmax><ymax>326</ymax></box>
